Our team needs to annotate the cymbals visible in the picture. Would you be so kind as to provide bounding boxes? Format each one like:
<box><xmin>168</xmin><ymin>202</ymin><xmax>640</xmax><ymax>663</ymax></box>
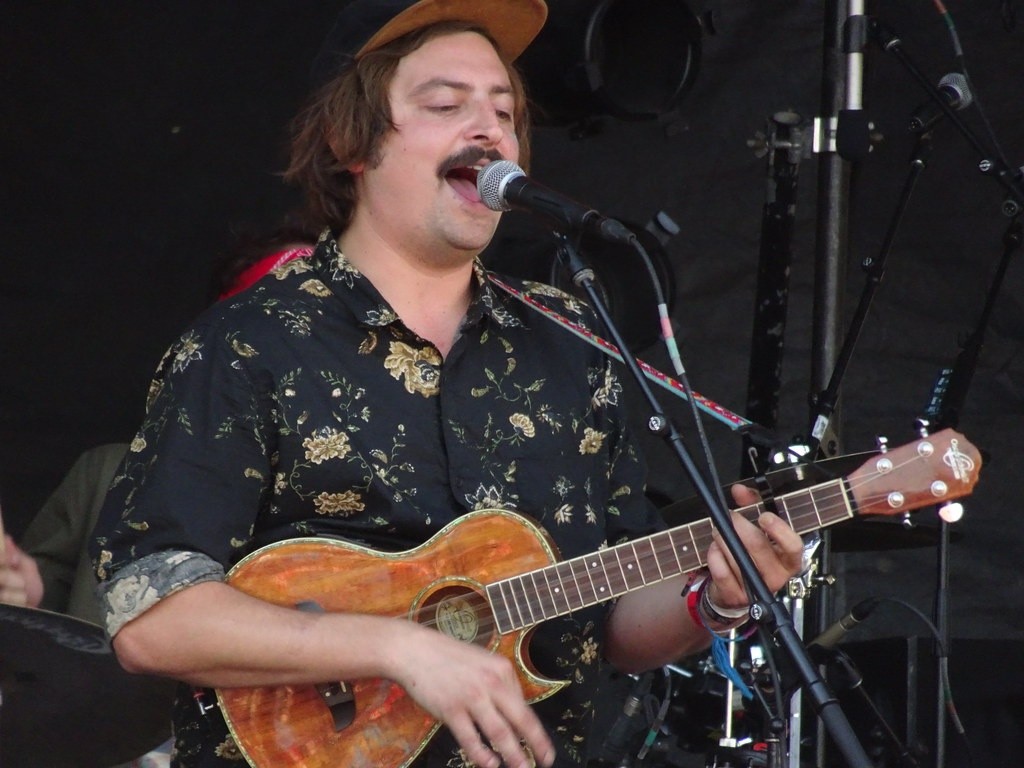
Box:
<box><xmin>641</xmin><ymin>501</ymin><xmax>964</xmax><ymax>550</ymax></box>
<box><xmin>1</xmin><ymin>602</ymin><xmax>184</xmax><ymax>768</ymax></box>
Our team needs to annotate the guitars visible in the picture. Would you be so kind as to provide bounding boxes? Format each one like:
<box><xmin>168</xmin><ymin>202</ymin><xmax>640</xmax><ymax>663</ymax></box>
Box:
<box><xmin>205</xmin><ymin>416</ymin><xmax>989</xmax><ymax>767</ymax></box>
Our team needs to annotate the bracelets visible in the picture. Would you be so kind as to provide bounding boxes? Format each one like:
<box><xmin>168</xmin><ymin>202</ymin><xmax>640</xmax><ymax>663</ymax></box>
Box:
<box><xmin>688</xmin><ymin>578</ymin><xmax>757</xmax><ymax>642</ymax></box>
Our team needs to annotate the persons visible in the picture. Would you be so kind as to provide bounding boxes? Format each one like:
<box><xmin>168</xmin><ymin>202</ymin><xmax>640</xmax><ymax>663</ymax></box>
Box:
<box><xmin>0</xmin><ymin>227</ymin><xmax>317</xmax><ymax>768</ymax></box>
<box><xmin>89</xmin><ymin>0</ymin><xmax>802</xmax><ymax>768</ymax></box>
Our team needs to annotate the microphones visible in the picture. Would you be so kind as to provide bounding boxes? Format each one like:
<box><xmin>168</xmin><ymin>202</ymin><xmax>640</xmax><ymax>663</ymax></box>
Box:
<box><xmin>477</xmin><ymin>159</ymin><xmax>636</xmax><ymax>245</ymax></box>
<box><xmin>805</xmin><ymin>601</ymin><xmax>875</xmax><ymax>658</ymax></box>
<box><xmin>908</xmin><ymin>72</ymin><xmax>973</xmax><ymax>134</ymax></box>
<box><xmin>599</xmin><ymin>672</ymin><xmax>654</xmax><ymax>763</ymax></box>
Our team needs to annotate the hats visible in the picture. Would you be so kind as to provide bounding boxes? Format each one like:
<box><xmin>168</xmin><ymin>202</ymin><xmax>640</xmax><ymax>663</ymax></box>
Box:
<box><xmin>354</xmin><ymin>0</ymin><xmax>548</xmax><ymax>68</ymax></box>
<box><xmin>218</xmin><ymin>246</ymin><xmax>312</xmax><ymax>300</ymax></box>
